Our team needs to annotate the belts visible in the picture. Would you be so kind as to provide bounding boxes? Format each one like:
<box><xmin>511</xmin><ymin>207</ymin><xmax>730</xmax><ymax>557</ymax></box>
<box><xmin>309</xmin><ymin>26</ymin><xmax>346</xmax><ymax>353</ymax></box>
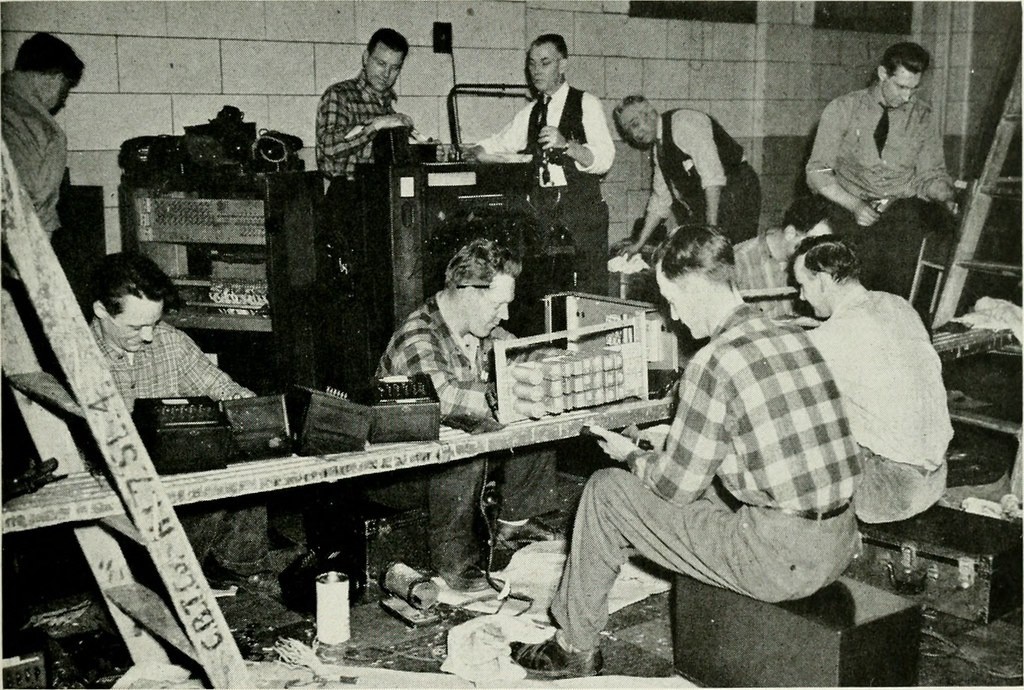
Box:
<box><xmin>797</xmin><ymin>495</ymin><xmax>854</xmax><ymax>521</ymax></box>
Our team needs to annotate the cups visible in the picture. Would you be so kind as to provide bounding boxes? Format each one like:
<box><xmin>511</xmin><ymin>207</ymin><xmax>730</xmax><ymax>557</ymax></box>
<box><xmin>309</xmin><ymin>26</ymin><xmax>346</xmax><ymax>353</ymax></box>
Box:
<box><xmin>316</xmin><ymin>571</ymin><xmax>350</xmax><ymax>644</ymax></box>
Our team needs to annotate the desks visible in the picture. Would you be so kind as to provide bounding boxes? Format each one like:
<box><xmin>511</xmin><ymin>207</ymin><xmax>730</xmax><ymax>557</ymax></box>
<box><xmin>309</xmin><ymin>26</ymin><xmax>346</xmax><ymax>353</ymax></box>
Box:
<box><xmin>0</xmin><ymin>325</ymin><xmax>1015</xmax><ymax>534</ymax></box>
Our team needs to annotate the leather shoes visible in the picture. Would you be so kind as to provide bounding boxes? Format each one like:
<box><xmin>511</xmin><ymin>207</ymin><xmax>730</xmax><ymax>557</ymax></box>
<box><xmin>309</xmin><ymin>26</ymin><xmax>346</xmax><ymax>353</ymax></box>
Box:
<box><xmin>509</xmin><ymin>637</ymin><xmax>604</xmax><ymax>678</ymax></box>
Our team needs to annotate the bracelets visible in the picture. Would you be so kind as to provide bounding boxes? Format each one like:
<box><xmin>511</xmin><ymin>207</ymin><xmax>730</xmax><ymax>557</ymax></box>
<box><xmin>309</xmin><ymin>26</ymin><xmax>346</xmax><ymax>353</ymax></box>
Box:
<box><xmin>561</xmin><ymin>137</ymin><xmax>569</xmax><ymax>153</ymax></box>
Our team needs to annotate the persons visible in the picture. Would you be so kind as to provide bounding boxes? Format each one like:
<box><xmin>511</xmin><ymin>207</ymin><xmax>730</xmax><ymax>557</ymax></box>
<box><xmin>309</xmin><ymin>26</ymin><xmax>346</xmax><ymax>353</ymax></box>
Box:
<box><xmin>86</xmin><ymin>253</ymin><xmax>287</xmax><ymax>602</ymax></box>
<box><xmin>315</xmin><ymin>29</ymin><xmax>443</xmax><ymax>196</ymax></box>
<box><xmin>372</xmin><ymin>239</ymin><xmax>565</xmax><ymax>590</ymax></box>
<box><xmin>612</xmin><ymin>95</ymin><xmax>762</xmax><ymax>262</ymax></box>
<box><xmin>0</xmin><ymin>30</ymin><xmax>86</xmax><ymax>280</ymax></box>
<box><xmin>806</xmin><ymin>41</ymin><xmax>955</xmax><ymax>239</ymax></box>
<box><xmin>510</xmin><ymin>196</ymin><xmax>953</xmax><ymax>678</ymax></box>
<box><xmin>448</xmin><ymin>34</ymin><xmax>615</xmax><ymax>301</ymax></box>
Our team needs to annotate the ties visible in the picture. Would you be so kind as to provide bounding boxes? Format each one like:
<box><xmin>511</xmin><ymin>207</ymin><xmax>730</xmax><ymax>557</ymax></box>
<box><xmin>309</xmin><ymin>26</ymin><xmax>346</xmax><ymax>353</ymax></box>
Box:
<box><xmin>540</xmin><ymin>96</ymin><xmax>552</xmax><ymax>129</ymax></box>
<box><xmin>656</xmin><ymin>138</ymin><xmax>677</xmax><ymax>204</ymax></box>
<box><xmin>873</xmin><ymin>101</ymin><xmax>894</xmax><ymax>159</ymax></box>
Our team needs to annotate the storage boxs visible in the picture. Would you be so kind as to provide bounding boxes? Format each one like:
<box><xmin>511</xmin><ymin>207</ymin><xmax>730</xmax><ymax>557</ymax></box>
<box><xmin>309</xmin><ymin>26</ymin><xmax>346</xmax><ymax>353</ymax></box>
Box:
<box><xmin>853</xmin><ymin>506</ymin><xmax>1023</xmax><ymax>623</ymax></box>
<box><xmin>675</xmin><ymin>569</ymin><xmax>920</xmax><ymax>688</ymax></box>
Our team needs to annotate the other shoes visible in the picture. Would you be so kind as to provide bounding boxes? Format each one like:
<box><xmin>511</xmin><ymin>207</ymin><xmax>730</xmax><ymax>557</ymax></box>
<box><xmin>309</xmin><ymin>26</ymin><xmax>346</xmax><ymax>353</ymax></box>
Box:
<box><xmin>431</xmin><ymin>559</ymin><xmax>488</xmax><ymax>592</ymax></box>
<box><xmin>491</xmin><ymin>518</ymin><xmax>567</xmax><ymax>552</ymax></box>
<box><xmin>204</xmin><ymin>558</ymin><xmax>282</xmax><ymax>595</ymax></box>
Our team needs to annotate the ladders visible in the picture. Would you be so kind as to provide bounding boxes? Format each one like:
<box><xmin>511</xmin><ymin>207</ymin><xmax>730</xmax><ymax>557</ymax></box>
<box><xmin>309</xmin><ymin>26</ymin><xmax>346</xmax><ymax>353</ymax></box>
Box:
<box><xmin>926</xmin><ymin>51</ymin><xmax>1023</xmax><ymax>520</ymax></box>
<box><xmin>1</xmin><ymin>132</ymin><xmax>251</xmax><ymax>689</ymax></box>
